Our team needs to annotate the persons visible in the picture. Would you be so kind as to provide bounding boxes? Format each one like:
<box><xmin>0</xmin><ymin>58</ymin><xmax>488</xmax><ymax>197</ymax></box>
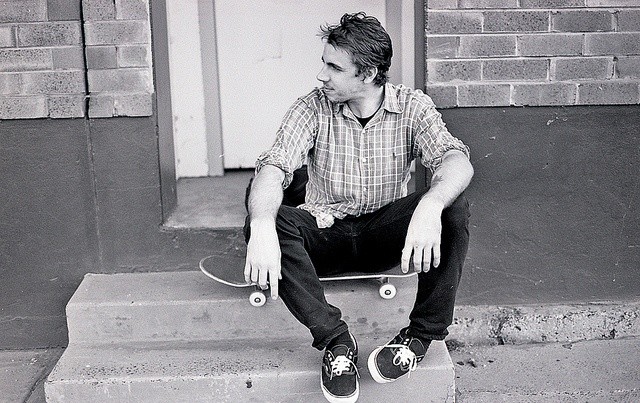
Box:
<box><xmin>244</xmin><ymin>11</ymin><xmax>474</xmax><ymax>403</ymax></box>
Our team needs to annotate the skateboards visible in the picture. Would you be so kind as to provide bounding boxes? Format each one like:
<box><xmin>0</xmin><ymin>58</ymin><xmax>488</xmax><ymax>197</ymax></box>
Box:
<box><xmin>199</xmin><ymin>255</ymin><xmax>418</xmax><ymax>307</ymax></box>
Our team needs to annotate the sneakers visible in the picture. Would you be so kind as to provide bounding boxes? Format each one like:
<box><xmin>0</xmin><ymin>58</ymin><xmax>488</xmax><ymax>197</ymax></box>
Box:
<box><xmin>320</xmin><ymin>331</ymin><xmax>360</xmax><ymax>403</ymax></box>
<box><xmin>367</xmin><ymin>327</ymin><xmax>427</xmax><ymax>384</ymax></box>
